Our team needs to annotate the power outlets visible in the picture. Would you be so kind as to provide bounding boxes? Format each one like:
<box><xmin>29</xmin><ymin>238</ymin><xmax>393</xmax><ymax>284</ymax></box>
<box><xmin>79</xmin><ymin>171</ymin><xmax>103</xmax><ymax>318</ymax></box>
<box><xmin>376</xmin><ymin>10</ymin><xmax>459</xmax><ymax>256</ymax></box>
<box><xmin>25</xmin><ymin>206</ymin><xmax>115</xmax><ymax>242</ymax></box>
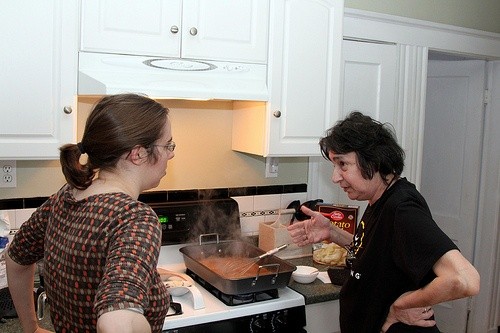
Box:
<box><xmin>0</xmin><ymin>160</ymin><xmax>17</xmax><ymax>188</ymax></box>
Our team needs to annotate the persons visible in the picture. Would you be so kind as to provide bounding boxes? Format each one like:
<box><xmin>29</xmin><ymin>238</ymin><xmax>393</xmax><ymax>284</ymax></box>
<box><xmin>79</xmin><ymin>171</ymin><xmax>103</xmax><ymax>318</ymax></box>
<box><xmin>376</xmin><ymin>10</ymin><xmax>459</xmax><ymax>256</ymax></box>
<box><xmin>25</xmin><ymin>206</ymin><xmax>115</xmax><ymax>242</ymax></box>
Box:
<box><xmin>4</xmin><ymin>93</ymin><xmax>177</xmax><ymax>333</ymax></box>
<box><xmin>286</xmin><ymin>111</ymin><xmax>481</xmax><ymax>333</ymax></box>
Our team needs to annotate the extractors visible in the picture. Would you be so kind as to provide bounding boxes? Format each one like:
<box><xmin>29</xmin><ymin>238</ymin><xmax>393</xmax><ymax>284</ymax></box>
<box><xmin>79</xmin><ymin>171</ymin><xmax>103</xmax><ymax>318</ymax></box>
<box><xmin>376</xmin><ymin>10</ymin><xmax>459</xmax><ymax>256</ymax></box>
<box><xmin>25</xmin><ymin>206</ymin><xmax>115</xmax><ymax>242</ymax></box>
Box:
<box><xmin>77</xmin><ymin>51</ymin><xmax>270</xmax><ymax>102</ymax></box>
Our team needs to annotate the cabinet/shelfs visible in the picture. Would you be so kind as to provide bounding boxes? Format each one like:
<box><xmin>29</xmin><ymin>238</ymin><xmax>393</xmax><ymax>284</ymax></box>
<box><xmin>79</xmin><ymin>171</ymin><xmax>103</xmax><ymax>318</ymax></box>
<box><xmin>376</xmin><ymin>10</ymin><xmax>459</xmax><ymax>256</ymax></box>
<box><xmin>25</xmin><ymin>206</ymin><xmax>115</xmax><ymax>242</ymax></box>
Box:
<box><xmin>78</xmin><ymin>0</ymin><xmax>269</xmax><ymax>102</ymax></box>
<box><xmin>233</xmin><ymin>0</ymin><xmax>342</xmax><ymax>158</ymax></box>
<box><xmin>0</xmin><ymin>0</ymin><xmax>79</xmax><ymax>161</ymax></box>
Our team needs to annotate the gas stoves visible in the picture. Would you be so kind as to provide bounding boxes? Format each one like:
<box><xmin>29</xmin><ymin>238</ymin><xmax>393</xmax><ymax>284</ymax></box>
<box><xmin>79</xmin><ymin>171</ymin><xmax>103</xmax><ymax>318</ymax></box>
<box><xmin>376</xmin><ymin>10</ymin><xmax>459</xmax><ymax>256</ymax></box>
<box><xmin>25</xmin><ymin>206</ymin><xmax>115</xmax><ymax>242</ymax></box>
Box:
<box><xmin>146</xmin><ymin>197</ymin><xmax>307</xmax><ymax>333</ymax></box>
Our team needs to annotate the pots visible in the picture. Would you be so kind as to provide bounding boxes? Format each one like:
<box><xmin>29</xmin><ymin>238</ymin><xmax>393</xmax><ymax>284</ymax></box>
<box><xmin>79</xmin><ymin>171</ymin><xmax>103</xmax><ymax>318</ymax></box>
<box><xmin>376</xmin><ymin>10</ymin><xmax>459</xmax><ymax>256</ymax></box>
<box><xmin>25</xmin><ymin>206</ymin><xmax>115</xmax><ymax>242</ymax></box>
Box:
<box><xmin>179</xmin><ymin>232</ymin><xmax>298</xmax><ymax>296</ymax></box>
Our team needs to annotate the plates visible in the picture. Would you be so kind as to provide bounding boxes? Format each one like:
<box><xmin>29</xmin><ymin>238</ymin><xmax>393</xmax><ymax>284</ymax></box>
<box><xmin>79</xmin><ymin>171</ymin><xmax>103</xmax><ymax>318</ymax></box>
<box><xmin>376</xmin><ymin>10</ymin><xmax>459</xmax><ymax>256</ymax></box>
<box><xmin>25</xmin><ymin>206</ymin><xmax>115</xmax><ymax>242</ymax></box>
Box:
<box><xmin>314</xmin><ymin>259</ymin><xmax>345</xmax><ymax>267</ymax></box>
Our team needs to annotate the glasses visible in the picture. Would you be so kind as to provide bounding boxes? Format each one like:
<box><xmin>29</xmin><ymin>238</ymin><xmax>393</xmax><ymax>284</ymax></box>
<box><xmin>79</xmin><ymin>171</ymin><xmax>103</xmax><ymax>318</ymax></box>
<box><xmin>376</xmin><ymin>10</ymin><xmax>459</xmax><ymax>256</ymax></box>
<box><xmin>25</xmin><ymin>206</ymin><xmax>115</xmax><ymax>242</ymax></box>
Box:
<box><xmin>125</xmin><ymin>141</ymin><xmax>175</xmax><ymax>160</ymax></box>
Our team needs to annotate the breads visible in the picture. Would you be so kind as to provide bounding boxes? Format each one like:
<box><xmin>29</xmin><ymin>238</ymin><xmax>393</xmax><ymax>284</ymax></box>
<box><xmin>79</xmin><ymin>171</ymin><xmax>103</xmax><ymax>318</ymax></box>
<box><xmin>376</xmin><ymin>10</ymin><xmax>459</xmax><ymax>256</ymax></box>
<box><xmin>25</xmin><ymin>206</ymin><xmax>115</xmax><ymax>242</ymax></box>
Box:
<box><xmin>312</xmin><ymin>242</ymin><xmax>349</xmax><ymax>266</ymax></box>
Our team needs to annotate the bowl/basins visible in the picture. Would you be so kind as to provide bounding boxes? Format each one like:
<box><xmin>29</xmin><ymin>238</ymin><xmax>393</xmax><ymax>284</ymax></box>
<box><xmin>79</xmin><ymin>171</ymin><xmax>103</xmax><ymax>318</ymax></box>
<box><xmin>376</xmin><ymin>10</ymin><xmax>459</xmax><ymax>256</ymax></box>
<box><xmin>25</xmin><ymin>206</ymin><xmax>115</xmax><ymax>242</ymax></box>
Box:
<box><xmin>291</xmin><ymin>266</ymin><xmax>319</xmax><ymax>284</ymax></box>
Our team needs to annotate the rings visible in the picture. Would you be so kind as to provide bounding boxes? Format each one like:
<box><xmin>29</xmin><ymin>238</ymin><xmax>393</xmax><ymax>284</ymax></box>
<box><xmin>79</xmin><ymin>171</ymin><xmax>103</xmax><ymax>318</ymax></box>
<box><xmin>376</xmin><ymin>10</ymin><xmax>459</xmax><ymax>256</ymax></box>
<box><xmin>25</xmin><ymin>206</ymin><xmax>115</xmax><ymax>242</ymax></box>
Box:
<box><xmin>424</xmin><ymin>308</ymin><xmax>427</xmax><ymax>311</ymax></box>
